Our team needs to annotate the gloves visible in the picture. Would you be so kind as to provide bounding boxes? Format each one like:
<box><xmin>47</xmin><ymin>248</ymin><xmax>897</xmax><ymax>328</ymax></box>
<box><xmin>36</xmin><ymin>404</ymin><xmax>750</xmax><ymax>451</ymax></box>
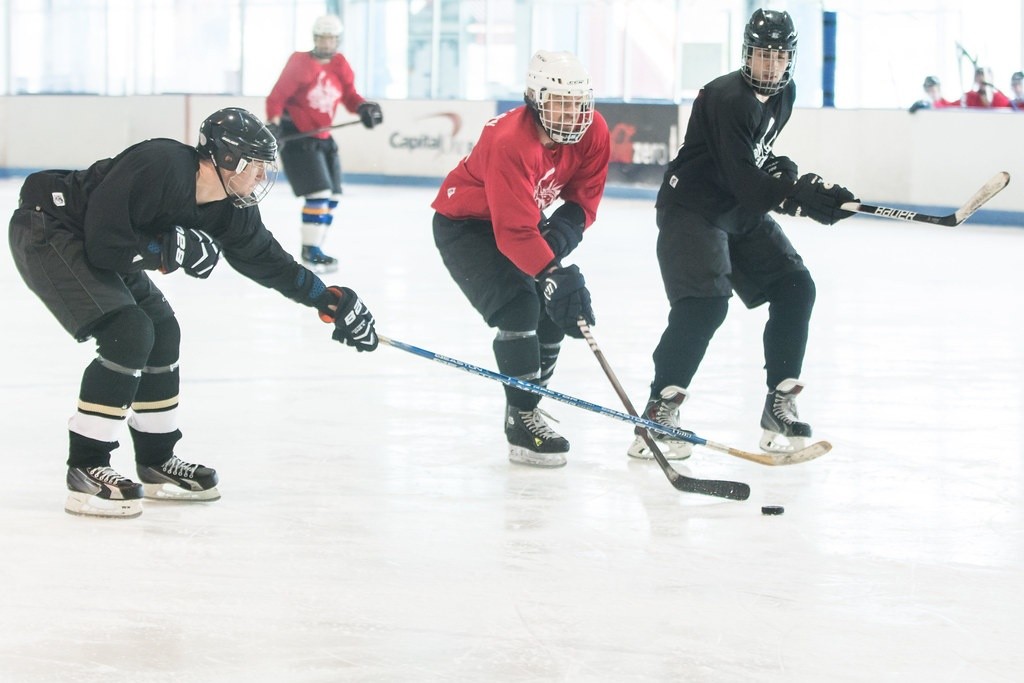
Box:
<box><xmin>536</xmin><ymin>263</ymin><xmax>597</xmax><ymax>339</ymax></box>
<box><xmin>265</xmin><ymin>120</ymin><xmax>289</xmax><ymax>157</ymax></box>
<box><xmin>910</xmin><ymin>98</ymin><xmax>929</xmax><ymax>114</ymax></box>
<box><xmin>761</xmin><ymin>158</ymin><xmax>800</xmax><ymax>214</ymax></box>
<box><xmin>357</xmin><ymin>102</ymin><xmax>383</xmax><ymax>129</ymax></box>
<box><xmin>538</xmin><ymin>213</ymin><xmax>587</xmax><ymax>269</ymax></box>
<box><xmin>783</xmin><ymin>173</ymin><xmax>860</xmax><ymax>225</ymax></box>
<box><xmin>318</xmin><ymin>283</ymin><xmax>382</xmax><ymax>352</ymax></box>
<box><xmin>160</xmin><ymin>224</ymin><xmax>221</xmax><ymax>279</ymax></box>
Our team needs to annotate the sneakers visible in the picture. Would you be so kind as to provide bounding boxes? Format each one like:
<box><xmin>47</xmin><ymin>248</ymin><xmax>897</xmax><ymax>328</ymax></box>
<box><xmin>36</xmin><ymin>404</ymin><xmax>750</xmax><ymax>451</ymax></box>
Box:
<box><xmin>759</xmin><ymin>384</ymin><xmax>811</xmax><ymax>451</ymax></box>
<box><xmin>627</xmin><ymin>382</ymin><xmax>695</xmax><ymax>460</ymax></box>
<box><xmin>135</xmin><ymin>451</ymin><xmax>224</xmax><ymax>501</ymax></box>
<box><xmin>64</xmin><ymin>463</ymin><xmax>146</xmax><ymax>518</ymax></box>
<box><xmin>301</xmin><ymin>245</ymin><xmax>341</xmax><ymax>274</ymax></box>
<box><xmin>504</xmin><ymin>400</ymin><xmax>573</xmax><ymax>468</ymax></box>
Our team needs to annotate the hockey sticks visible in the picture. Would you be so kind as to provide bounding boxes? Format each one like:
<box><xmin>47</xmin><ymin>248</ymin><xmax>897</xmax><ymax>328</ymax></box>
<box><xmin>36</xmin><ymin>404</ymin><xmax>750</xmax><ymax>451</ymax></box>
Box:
<box><xmin>841</xmin><ymin>172</ymin><xmax>1011</xmax><ymax>228</ymax></box>
<box><xmin>374</xmin><ymin>331</ymin><xmax>834</xmax><ymax>465</ymax></box>
<box><xmin>273</xmin><ymin>120</ymin><xmax>360</xmax><ymax>146</ymax></box>
<box><xmin>577</xmin><ymin>314</ymin><xmax>750</xmax><ymax>501</ymax></box>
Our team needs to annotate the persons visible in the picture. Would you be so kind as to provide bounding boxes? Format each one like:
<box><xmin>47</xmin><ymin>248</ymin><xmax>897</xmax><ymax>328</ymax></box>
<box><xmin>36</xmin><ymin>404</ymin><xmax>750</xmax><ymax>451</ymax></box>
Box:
<box><xmin>909</xmin><ymin>76</ymin><xmax>961</xmax><ymax>113</ymax></box>
<box><xmin>6</xmin><ymin>106</ymin><xmax>379</xmax><ymax>519</ymax></box>
<box><xmin>627</xmin><ymin>8</ymin><xmax>860</xmax><ymax>460</ymax></box>
<box><xmin>1009</xmin><ymin>72</ymin><xmax>1023</xmax><ymax>110</ymax></box>
<box><xmin>962</xmin><ymin>67</ymin><xmax>1010</xmax><ymax>108</ymax></box>
<box><xmin>263</xmin><ymin>14</ymin><xmax>383</xmax><ymax>273</ymax></box>
<box><xmin>430</xmin><ymin>46</ymin><xmax>611</xmax><ymax>469</ymax></box>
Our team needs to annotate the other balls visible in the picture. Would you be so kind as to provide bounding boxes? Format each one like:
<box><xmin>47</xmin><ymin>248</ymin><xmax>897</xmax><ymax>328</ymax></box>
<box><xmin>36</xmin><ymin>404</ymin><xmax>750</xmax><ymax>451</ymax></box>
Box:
<box><xmin>762</xmin><ymin>506</ymin><xmax>784</xmax><ymax>515</ymax></box>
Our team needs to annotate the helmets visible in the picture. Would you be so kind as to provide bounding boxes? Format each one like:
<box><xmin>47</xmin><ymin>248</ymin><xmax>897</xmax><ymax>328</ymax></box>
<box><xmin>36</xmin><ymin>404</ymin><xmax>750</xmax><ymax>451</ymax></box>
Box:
<box><xmin>741</xmin><ymin>9</ymin><xmax>802</xmax><ymax>97</ymax></box>
<box><xmin>311</xmin><ymin>14</ymin><xmax>343</xmax><ymax>59</ymax></box>
<box><xmin>196</xmin><ymin>107</ymin><xmax>280</xmax><ymax>209</ymax></box>
<box><xmin>924</xmin><ymin>77</ymin><xmax>940</xmax><ymax>89</ymax></box>
<box><xmin>522</xmin><ymin>51</ymin><xmax>595</xmax><ymax>144</ymax></box>
